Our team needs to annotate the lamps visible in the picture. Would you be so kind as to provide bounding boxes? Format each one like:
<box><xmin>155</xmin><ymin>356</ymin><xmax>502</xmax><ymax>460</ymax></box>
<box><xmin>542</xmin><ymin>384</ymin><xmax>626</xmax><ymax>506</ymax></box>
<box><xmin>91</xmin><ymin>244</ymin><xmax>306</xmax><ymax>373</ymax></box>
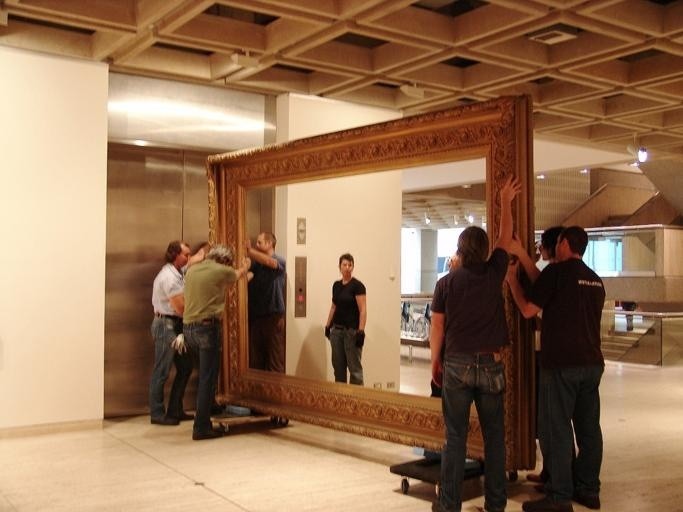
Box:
<box><xmin>638</xmin><ymin>146</ymin><xmax>649</xmax><ymax>162</ymax></box>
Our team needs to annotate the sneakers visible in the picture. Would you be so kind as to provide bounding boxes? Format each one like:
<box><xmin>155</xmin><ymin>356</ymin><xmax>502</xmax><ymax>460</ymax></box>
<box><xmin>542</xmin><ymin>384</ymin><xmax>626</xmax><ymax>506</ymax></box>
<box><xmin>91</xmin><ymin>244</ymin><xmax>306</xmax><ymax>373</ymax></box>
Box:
<box><xmin>151</xmin><ymin>403</ymin><xmax>226</xmax><ymax>440</ymax></box>
<box><xmin>522</xmin><ymin>473</ymin><xmax>600</xmax><ymax>512</ymax></box>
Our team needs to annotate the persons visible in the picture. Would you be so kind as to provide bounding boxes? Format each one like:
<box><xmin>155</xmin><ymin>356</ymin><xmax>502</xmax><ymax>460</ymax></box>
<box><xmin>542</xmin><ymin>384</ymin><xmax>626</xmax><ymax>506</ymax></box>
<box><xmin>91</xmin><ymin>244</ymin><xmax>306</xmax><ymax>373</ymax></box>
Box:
<box><xmin>427</xmin><ymin>171</ymin><xmax>521</xmax><ymax>512</ymax></box>
<box><xmin>325</xmin><ymin>253</ymin><xmax>368</xmax><ymax>385</ymax></box>
<box><xmin>149</xmin><ymin>239</ymin><xmax>194</xmax><ymax>425</ymax></box>
<box><xmin>429</xmin><ymin>255</ymin><xmax>462</xmax><ymax>396</ymax></box>
<box><xmin>525</xmin><ymin>225</ymin><xmax>565</xmax><ymax>495</ymax></box>
<box><xmin>505</xmin><ymin>226</ymin><xmax>605</xmax><ymax>512</ymax></box>
<box><xmin>242</xmin><ymin>230</ymin><xmax>288</xmax><ymax>427</ymax></box>
<box><xmin>183</xmin><ymin>244</ymin><xmax>253</xmax><ymax>442</ymax></box>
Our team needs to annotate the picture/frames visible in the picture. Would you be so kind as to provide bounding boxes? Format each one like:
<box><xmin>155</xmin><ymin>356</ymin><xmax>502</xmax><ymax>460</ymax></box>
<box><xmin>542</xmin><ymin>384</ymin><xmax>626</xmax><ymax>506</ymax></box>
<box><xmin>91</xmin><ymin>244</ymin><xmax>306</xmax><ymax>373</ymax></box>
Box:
<box><xmin>204</xmin><ymin>93</ymin><xmax>538</xmax><ymax>471</ymax></box>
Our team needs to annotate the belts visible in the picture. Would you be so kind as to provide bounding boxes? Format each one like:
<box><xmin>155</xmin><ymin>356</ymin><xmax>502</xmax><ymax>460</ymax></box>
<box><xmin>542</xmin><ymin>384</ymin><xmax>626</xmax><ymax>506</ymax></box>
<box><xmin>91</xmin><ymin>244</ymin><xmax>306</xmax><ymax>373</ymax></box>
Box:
<box><xmin>157</xmin><ymin>313</ymin><xmax>179</xmax><ymax>319</ymax></box>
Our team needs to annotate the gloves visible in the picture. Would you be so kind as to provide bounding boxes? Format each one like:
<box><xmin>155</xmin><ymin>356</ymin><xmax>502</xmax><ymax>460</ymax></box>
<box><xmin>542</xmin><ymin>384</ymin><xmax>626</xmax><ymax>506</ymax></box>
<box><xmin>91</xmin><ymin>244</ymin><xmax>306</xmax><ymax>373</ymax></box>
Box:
<box><xmin>170</xmin><ymin>334</ymin><xmax>187</xmax><ymax>355</ymax></box>
<box><xmin>325</xmin><ymin>326</ymin><xmax>331</xmax><ymax>337</ymax></box>
<box><xmin>355</xmin><ymin>329</ymin><xmax>365</xmax><ymax>346</ymax></box>
